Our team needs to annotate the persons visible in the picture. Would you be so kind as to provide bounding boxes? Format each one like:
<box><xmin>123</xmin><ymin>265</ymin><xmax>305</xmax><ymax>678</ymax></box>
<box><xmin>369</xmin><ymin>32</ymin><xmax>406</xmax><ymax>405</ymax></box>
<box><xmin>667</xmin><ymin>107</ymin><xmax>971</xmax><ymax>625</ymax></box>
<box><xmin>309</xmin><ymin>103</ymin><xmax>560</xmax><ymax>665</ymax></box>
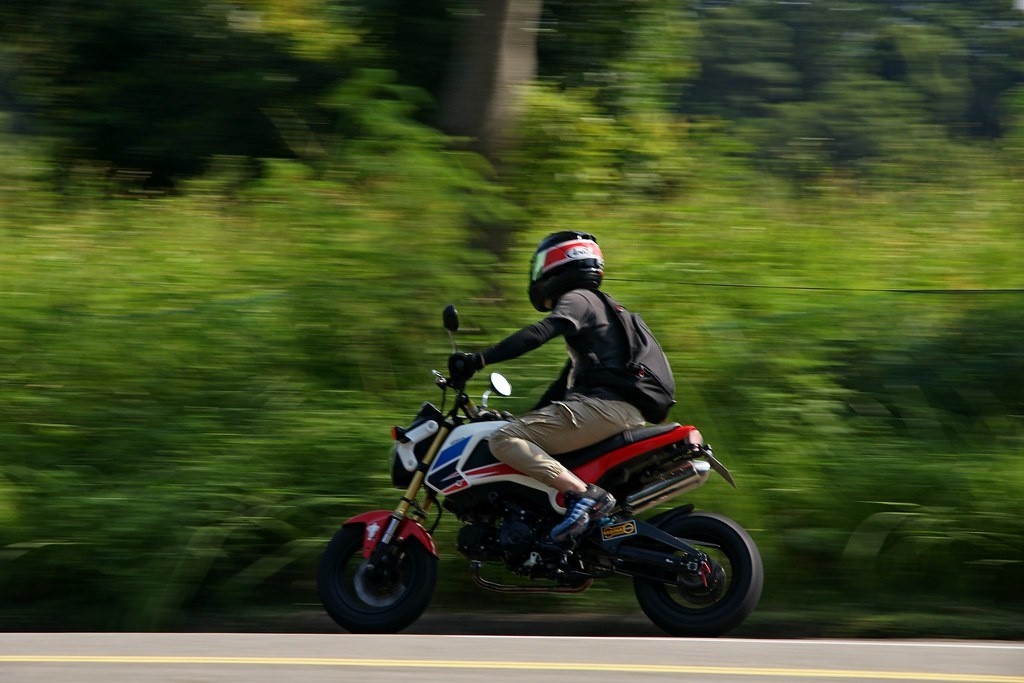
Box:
<box><xmin>448</xmin><ymin>231</ymin><xmax>676</xmax><ymax>540</ymax></box>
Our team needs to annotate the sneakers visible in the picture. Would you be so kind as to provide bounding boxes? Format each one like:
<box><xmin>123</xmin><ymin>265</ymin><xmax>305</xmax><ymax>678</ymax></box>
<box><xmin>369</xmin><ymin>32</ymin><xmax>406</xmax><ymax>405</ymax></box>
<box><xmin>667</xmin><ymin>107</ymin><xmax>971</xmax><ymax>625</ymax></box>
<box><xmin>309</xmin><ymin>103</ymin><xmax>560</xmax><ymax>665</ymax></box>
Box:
<box><xmin>550</xmin><ymin>483</ymin><xmax>616</xmax><ymax>540</ymax></box>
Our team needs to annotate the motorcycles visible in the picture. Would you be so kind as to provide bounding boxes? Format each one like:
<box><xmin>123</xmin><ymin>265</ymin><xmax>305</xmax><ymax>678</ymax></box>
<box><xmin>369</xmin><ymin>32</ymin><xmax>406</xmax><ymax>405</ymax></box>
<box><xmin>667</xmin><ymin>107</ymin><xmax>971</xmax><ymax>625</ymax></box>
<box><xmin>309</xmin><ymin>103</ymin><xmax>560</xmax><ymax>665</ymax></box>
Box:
<box><xmin>319</xmin><ymin>302</ymin><xmax>764</xmax><ymax>637</ymax></box>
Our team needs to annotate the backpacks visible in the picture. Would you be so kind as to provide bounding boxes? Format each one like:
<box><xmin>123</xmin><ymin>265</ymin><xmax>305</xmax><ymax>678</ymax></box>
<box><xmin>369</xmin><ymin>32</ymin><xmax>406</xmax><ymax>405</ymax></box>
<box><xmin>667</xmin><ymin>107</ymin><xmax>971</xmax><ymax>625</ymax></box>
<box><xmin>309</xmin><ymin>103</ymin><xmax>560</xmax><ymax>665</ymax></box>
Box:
<box><xmin>568</xmin><ymin>289</ymin><xmax>676</xmax><ymax>425</ymax></box>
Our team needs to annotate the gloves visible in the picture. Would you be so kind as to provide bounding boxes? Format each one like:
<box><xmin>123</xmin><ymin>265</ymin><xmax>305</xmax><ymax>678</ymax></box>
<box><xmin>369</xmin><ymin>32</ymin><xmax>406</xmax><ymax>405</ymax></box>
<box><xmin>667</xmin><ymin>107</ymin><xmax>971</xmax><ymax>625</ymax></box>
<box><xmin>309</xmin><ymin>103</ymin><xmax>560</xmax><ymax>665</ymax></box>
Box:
<box><xmin>476</xmin><ymin>405</ymin><xmax>513</xmax><ymax>419</ymax></box>
<box><xmin>448</xmin><ymin>351</ymin><xmax>484</xmax><ymax>386</ymax></box>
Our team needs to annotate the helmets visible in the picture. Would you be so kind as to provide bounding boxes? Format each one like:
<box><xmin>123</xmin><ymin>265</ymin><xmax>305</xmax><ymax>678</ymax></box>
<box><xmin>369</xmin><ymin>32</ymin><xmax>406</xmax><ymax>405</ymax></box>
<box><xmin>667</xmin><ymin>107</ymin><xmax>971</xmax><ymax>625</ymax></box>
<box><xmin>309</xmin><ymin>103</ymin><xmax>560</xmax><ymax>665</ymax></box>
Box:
<box><xmin>528</xmin><ymin>230</ymin><xmax>604</xmax><ymax>312</ymax></box>
